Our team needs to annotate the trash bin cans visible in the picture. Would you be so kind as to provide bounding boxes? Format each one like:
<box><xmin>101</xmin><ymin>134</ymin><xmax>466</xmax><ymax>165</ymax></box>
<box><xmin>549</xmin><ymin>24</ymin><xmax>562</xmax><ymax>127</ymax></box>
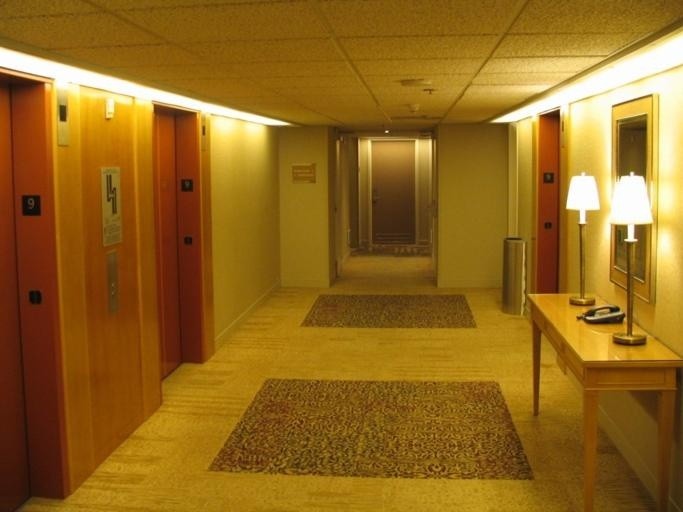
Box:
<box><xmin>502</xmin><ymin>237</ymin><xmax>527</xmax><ymax>316</ymax></box>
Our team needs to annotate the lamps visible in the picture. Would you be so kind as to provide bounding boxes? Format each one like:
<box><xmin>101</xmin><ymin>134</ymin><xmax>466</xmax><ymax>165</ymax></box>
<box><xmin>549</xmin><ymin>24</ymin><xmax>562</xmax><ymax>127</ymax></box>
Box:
<box><xmin>607</xmin><ymin>171</ymin><xmax>654</xmax><ymax>347</ymax></box>
<box><xmin>565</xmin><ymin>171</ymin><xmax>599</xmax><ymax>307</ymax></box>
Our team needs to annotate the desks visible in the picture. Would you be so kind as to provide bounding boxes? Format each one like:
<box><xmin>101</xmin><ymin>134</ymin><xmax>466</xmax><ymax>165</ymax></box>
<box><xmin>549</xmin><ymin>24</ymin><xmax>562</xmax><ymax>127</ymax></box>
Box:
<box><xmin>523</xmin><ymin>292</ymin><xmax>681</xmax><ymax>512</ymax></box>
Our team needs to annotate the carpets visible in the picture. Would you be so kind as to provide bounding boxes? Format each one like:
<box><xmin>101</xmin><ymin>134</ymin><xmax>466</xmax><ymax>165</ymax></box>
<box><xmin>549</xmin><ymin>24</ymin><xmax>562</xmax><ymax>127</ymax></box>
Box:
<box><xmin>206</xmin><ymin>377</ymin><xmax>534</xmax><ymax>482</ymax></box>
<box><xmin>301</xmin><ymin>291</ymin><xmax>475</xmax><ymax>330</ymax></box>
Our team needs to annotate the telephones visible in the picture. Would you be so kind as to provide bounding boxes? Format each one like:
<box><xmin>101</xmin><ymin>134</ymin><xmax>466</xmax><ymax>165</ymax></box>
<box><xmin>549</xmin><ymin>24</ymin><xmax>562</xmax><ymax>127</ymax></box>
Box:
<box><xmin>583</xmin><ymin>305</ymin><xmax>625</xmax><ymax>323</ymax></box>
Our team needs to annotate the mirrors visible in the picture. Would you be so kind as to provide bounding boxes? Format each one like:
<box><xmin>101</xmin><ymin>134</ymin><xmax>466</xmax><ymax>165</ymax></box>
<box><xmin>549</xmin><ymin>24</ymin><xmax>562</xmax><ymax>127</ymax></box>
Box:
<box><xmin>608</xmin><ymin>90</ymin><xmax>658</xmax><ymax>304</ymax></box>
